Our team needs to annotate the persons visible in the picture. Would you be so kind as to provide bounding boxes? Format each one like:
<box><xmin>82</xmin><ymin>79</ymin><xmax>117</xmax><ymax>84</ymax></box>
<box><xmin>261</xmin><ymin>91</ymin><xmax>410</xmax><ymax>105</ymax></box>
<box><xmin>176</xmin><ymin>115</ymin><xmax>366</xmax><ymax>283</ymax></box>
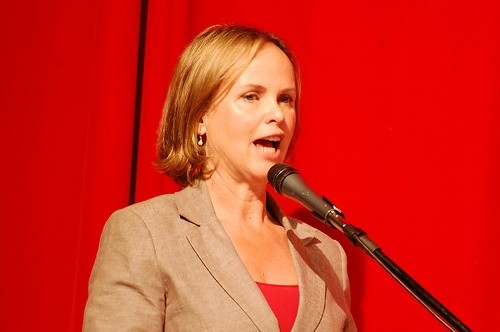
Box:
<box><xmin>82</xmin><ymin>24</ymin><xmax>360</xmax><ymax>332</ymax></box>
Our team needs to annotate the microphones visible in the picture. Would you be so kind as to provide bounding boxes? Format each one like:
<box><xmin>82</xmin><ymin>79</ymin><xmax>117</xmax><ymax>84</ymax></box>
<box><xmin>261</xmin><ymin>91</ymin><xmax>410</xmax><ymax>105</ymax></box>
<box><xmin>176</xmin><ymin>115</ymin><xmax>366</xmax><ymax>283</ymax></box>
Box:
<box><xmin>267</xmin><ymin>163</ymin><xmax>357</xmax><ymax>238</ymax></box>
